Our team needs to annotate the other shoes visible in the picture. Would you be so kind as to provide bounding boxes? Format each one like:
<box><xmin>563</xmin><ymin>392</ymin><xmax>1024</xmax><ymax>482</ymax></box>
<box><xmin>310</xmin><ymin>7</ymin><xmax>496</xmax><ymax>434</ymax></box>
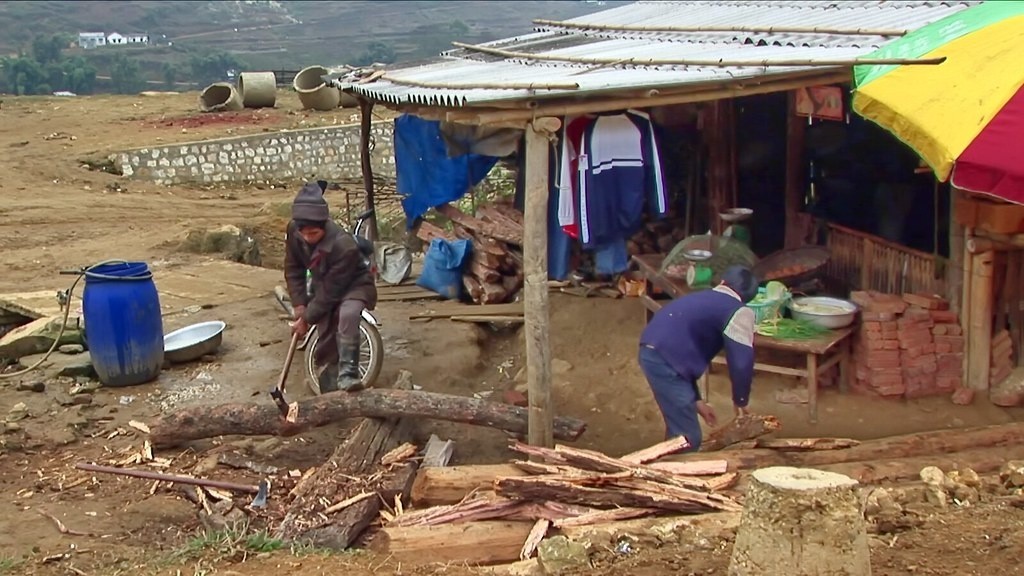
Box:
<box><xmin>337</xmin><ymin>373</ymin><xmax>364</xmax><ymax>392</ymax></box>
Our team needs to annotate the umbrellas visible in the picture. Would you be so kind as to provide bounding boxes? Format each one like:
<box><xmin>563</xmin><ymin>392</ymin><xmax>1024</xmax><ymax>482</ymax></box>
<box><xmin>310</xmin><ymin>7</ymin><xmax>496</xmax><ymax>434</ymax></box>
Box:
<box><xmin>850</xmin><ymin>1</ymin><xmax>1024</xmax><ymax>206</ymax></box>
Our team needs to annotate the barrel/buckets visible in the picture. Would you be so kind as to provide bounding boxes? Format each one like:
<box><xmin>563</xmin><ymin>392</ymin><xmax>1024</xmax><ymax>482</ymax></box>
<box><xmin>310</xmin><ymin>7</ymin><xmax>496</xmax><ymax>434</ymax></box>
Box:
<box><xmin>82</xmin><ymin>263</ymin><xmax>164</xmax><ymax>385</ymax></box>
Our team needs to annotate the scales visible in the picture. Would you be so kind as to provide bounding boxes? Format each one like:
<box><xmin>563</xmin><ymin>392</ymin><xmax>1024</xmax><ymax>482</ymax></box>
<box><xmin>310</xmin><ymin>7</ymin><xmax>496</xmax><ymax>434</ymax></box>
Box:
<box><xmin>683</xmin><ymin>249</ymin><xmax>713</xmax><ymax>290</ymax></box>
<box><xmin>718</xmin><ymin>207</ymin><xmax>754</xmax><ymax>250</ymax></box>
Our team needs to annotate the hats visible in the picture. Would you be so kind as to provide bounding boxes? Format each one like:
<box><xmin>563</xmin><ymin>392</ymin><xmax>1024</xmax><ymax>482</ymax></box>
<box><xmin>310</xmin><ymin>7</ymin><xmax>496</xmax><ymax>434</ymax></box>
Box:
<box><xmin>291</xmin><ymin>182</ymin><xmax>329</xmax><ymax>220</ymax></box>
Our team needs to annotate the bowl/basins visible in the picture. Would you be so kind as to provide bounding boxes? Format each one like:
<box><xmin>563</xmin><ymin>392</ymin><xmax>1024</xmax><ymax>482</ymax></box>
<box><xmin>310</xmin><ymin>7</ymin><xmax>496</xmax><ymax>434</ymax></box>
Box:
<box><xmin>745</xmin><ymin>287</ymin><xmax>791</xmax><ymax>325</ymax></box>
<box><xmin>787</xmin><ymin>297</ymin><xmax>856</xmax><ymax>328</ymax></box>
<box><xmin>164</xmin><ymin>321</ymin><xmax>225</xmax><ymax>362</ymax></box>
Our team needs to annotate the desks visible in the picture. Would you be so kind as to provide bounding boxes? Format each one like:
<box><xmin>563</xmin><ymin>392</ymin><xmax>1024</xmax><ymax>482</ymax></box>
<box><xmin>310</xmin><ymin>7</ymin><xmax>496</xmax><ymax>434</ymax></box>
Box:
<box><xmin>696</xmin><ymin>323</ymin><xmax>858</xmax><ymax>422</ymax></box>
<box><xmin>632</xmin><ymin>253</ymin><xmax>706</xmax><ymax>326</ymax></box>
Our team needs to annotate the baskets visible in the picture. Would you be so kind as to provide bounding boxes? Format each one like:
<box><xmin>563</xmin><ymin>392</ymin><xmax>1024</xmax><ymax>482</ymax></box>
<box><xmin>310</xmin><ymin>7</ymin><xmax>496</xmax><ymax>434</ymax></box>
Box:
<box><xmin>744</xmin><ymin>287</ymin><xmax>791</xmax><ymax>324</ymax></box>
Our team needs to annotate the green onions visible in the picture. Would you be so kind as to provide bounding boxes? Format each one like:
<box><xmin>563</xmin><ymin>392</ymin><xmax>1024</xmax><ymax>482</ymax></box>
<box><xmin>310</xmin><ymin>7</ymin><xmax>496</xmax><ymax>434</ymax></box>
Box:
<box><xmin>756</xmin><ymin>317</ymin><xmax>834</xmax><ymax>339</ymax></box>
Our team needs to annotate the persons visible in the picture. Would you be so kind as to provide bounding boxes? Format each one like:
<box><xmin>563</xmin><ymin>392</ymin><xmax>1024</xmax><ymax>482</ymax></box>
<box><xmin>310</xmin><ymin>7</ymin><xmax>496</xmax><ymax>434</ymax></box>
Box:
<box><xmin>637</xmin><ymin>264</ymin><xmax>759</xmax><ymax>456</ymax></box>
<box><xmin>284</xmin><ymin>181</ymin><xmax>378</xmax><ymax>395</ymax></box>
